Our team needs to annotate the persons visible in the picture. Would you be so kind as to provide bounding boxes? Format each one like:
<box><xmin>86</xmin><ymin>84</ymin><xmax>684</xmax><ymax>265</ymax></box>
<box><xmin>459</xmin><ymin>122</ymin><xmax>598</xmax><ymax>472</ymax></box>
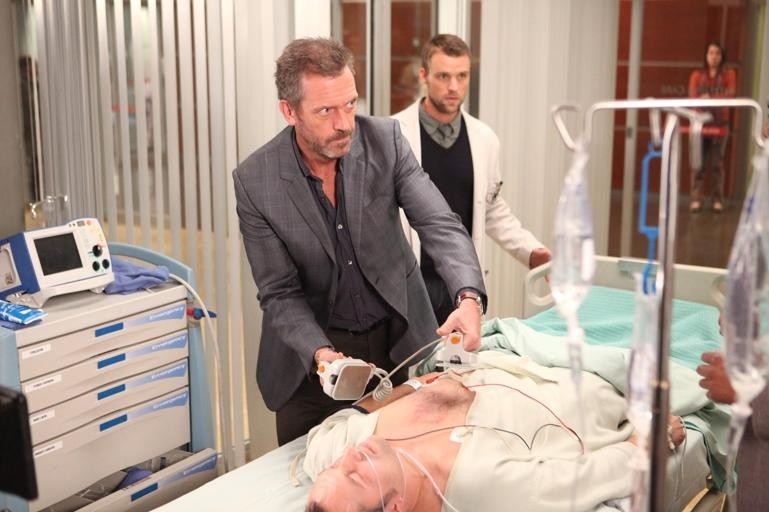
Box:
<box><xmin>303</xmin><ymin>351</ymin><xmax>686</xmax><ymax>512</ymax></box>
<box><xmin>688</xmin><ymin>40</ymin><xmax>737</xmax><ymax>212</ymax></box>
<box><xmin>389</xmin><ymin>34</ymin><xmax>552</xmax><ymax>333</ymax></box>
<box><xmin>232</xmin><ymin>36</ymin><xmax>487</xmax><ymax>447</ymax></box>
<box><xmin>697</xmin><ymin>307</ymin><xmax>769</xmax><ymax>512</ymax></box>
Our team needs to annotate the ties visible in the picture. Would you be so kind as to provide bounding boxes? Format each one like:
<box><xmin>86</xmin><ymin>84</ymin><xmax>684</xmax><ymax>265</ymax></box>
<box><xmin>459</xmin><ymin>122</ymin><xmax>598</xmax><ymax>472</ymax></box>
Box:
<box><xmin>437</xmin><ymin>124</ymin><xmax>452</xmax><ymax>148</ymax></box>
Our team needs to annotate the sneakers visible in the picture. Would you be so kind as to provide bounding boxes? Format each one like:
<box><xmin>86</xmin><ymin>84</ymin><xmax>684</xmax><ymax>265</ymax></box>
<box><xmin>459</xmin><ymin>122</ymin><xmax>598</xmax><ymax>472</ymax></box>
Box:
<box><xmin>689</xmin><ymin>202</ymin><xmax>724</xmax><ymax>212</ymax></box>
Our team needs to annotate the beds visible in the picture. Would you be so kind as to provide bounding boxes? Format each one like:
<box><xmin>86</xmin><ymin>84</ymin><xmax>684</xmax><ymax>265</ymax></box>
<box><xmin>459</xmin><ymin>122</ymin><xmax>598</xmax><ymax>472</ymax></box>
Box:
<box><xmin>133</xmin><ymin>247</ymin><xmax>739</xmax><ymax>510</ymax></box>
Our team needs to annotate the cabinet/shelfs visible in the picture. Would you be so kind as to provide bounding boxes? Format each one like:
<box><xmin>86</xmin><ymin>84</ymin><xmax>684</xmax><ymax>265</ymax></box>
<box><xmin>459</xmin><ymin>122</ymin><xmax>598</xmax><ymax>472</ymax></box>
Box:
<box><xmin>0</xmin><ymin>284</ymin><xmax>190</xmax><ymax>512</ymax></box>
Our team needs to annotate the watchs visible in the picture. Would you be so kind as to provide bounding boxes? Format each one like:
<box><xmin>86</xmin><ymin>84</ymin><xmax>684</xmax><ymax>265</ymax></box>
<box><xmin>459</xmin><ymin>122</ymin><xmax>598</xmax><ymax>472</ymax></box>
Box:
<box><xmin>456</xmin><ymin>291</ymin><xmax>484</xmax><ymax>316</ymax></box>
